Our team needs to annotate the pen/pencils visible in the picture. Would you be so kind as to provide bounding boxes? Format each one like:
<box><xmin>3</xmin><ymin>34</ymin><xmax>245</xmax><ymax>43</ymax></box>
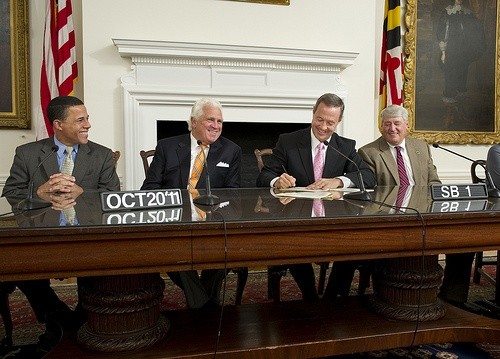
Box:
<box><xmin>282</xmin><ymin>165</ymin><xmax>295</xmax><ymax>188</ymax></box>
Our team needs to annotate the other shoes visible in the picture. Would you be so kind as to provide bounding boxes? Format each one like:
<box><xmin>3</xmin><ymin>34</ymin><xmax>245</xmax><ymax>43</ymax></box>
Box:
<box><xmin>38</xmin><ymin>303</ymin><xmax>71</xmax><ymax>344</ymax></box>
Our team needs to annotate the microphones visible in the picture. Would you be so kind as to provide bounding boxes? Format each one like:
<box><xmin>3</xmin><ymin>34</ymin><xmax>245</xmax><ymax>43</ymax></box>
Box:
<box><xmin>192</xmin><ymin>139</ymin><xmax>221</xmax><ymax>206</ymax></box>
<box><xmin>323</xmin><ymin>139</ymin><xmax>372</xmax><ymax>202</ymax></box>
<box><xmin>18</xmin><ymin>144</ymin><xmax>60</xmax><ymax>210</ymax></box>
<box><xmin>433</xmin><ymin>141</ymin><xmax>500</xmax><ymax>197</ymax></box>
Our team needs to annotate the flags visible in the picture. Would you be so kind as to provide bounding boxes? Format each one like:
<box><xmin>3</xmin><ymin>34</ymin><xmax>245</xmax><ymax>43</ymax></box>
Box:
<box><xmin>37</xmin><ymin>0</ymin><xmax>78</xmax><ymax>142</ymax></box>
<box><xmin>378</xmin><ymin>0</ymin><xmax>406</xmax><ymax>133</ymax></box>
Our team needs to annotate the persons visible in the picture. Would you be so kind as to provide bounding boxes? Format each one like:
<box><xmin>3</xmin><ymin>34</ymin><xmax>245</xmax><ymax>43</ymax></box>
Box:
<box><xmin>180</xmin><ymin>189</ymin><xmax>242</xmax><ymax>221</ymax></box>
<box><xmin>358</xmin><ymin>105</ymin><xmax>476</xmax><ymax>307</ymax></box>
<box><xmin>2</xmin><ymin>95</ymin><xmax>120</xmax><ymax>346</ymax></box>
<box><xmin>140</xmin><ymin>98</ymin><xmax>242</xmax><ymax>308</ymax></box>
<box><xmin>486</xmin><ymin>143</ymin><xmax>500</xmax><ymax>191</ymax></box>
<box><xmin>256</xmin><ymin>93</ymin><xmax>377</xmax><ymax>300</ymax></box>
<box><xmin>360</xmin><ymin>187</ymin><xmax>433</xmax><ymax>215</ymax></box>
<box><xmin>6</xmin><ymin>197</ymin><xmax>104</xmax><ymax>227</ymax></box>
<box><xmin>259</xmin><ymin>189</ymin><xmax>367</xmax><ymax>217</ymax></box>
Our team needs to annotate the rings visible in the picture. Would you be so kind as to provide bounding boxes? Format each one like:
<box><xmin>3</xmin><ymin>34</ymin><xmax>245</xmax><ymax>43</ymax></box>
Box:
<box><xmin>64</xmin><ymin>187</ymin><xmax>67</xmax><ymax>189</ymax></box>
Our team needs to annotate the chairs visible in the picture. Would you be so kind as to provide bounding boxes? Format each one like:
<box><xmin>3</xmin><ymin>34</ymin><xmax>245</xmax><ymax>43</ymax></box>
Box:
<box><xmin>255</xmin><ymin>148</ymin><xmax>332</xmax><ymax>300</ymax></box>
<box><xmin>141</xmin><ymin>147</ymin><xmax>250</xmax><ymax>304</ymax></box>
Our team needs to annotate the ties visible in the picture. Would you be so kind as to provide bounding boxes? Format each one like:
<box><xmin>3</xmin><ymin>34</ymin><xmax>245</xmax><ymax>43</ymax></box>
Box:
<box><xmin>189</xmin><ymin>189</ymin><xmax>206</xmax><ymax>221</ymax></box>
<box><xmin>61</xmin><ymin>147</ymin><xmax>74</xmax><ymax>176</ymax></box>
<box><xmin>313</xmin><ymin>199</ymin><xmax>322</xmax><ymax>217</ymax></box>
<box><xmin>188</xmin><ymin>145</ymin><xmax>207</xmax><ymax>189</ymax></box>
<box><xmin>313</xmin><ymin>143</ymin><xmax>324</xmax><ymax>182</ymax></box>
<box><xmin>62</xmin><ymin>206</ymin><xmax>76</xmax><ymax>225</ymax></box>
<box><xmin>395</xmin><ymin>147</ymin><xmax>410</xmax><ymax>185</ymax></box>
<box><xmin>395</xmin><ymin>185</ymin><xmax>409</xmax><ymax>214</ymax></box>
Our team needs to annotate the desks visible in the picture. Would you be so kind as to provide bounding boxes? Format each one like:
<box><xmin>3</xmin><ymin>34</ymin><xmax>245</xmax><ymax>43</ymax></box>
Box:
<box><xmin>0</xmin><ymin>182</ymin><xmax>500</xmax><ymax>359</ymax></box>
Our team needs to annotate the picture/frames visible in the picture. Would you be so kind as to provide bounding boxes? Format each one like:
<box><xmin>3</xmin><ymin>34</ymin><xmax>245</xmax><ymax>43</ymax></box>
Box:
<box><xmin>401</xmin><ymin>0</ymin><xmax>500</xmax><ymax>147</ymax></box>
<box><xmin>0</xmin><ymin>0</ymin><xmax>32</xmax><ymax>131</ymax></box>
